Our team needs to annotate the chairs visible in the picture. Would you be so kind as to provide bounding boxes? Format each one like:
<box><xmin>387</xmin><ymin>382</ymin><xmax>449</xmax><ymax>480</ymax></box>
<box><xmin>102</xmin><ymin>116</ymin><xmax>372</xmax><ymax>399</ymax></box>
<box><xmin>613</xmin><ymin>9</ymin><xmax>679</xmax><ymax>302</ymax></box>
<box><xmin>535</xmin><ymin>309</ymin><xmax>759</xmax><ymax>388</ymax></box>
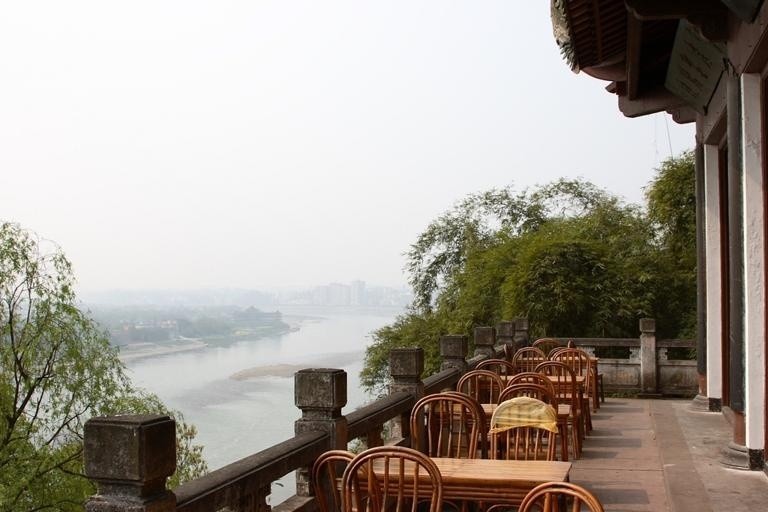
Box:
<box><xmin>311</xmin><ymin>451</ymin><xmax>378</xmax><ymax>508</ymax></box>
<box><xmin>517</xmin><ymin>482</ymin><xmax>603</xmax><ymax>512</ymax></box>
<box><xmin>338</xmin><ymin>445</ymin><xmax>445</xmax><ymax>511</ymax></box>
<box><xmin>410</xmin><ymin>347</ymin><xmax>601</xmax><ymax>461</ymax></box>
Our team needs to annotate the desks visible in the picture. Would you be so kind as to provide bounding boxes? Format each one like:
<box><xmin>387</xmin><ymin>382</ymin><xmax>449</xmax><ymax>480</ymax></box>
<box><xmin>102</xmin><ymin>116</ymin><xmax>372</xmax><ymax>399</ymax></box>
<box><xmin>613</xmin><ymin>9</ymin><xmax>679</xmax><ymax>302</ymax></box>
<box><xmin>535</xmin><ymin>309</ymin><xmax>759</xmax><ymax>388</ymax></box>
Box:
<box><xmin>335</xmin><ymin>457</ymin><xmax>573</xmax><ymax>512</ymax></box>
